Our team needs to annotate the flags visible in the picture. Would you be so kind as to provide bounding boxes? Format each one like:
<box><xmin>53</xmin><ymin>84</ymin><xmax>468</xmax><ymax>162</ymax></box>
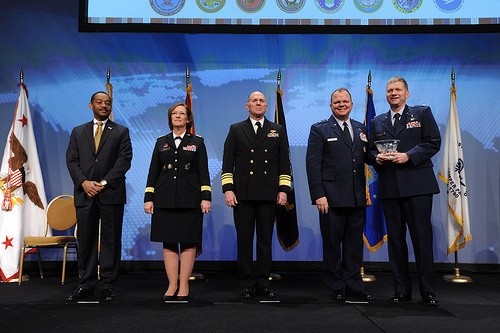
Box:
<box><xmin>183</xmin><ymin>92</ymin><xmax>195</xmax><ymax>135</ymax></box>
<box><xmin>107</xmin><ymin>84</ymin><xmax>114</xmax><ymax>121</ymax></box>
<box><xmin>275</xmin><ymin>90</ymin><xmax>300</xmax><ymax>252</ymax></box>
<box><xmin>437</xmin><ymin>87</ymin><xmax>473</xmax><ymax>256</ymax></box>
<box><xmin>0</xmin><ymin>84</ymin><xmax>54</xmax><ymax>283</ymax></box>
<box><xmin>364</xmin><ymin>86</ymin><xmax>388</xmax><ymax>253</ymax></box>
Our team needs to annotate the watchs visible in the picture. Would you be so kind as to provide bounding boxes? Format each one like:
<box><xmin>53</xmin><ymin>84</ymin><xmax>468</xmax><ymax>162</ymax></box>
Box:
<box><xmin>101</xmin><ymin>179</ymin><xmax>108</xmax><ymax>188</ymax></box>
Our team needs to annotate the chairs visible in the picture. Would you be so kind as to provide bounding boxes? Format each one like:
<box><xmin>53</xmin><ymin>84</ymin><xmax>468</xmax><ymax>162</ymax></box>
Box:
<box><xmin>18</xmin><ymin>195</ymin><xmax>78</xmax><ymax>286</ymax></box>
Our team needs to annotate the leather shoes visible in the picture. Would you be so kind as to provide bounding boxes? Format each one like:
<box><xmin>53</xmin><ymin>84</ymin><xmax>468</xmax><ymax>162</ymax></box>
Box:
<box><xmin>238</xmin><ymin>287</ymin><xmax>254</xmax><ymax>298</ymax></box>
<box><xmin>390</xmin><ymin>293</ymin><xmax>412</xmax><ymax>302</ymax></box>
<box><xmin>262</xmin><ymin>286</ymin><xmax>280</xmax><ymax>298</ymax></box>
<box><xmin>97</xmin><ymin>288</ymin><xmax>117</xmax><ymax>301</ymax></box>
<box><xmin>65</xmin><ymin>286</ymin><xmax>94</xmax><ymax>302</ymax></box>
<box><xmin>327</xmin><ymin>287</ymin><xmax>346</xmax><ymax>300</ymax></box>
<box><xmin>421</xmin><ymin>291</ymin><xmax>440</xmax><ymax>306</ymax></box>
<box><xmin>351</xmin><ymin>288</ymin><xmax>373</xmax><ymax>299</ymax></box>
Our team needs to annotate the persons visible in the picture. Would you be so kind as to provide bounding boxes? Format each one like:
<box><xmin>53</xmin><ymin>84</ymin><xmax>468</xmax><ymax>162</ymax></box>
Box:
<box><xmin>305</xmin><ymin>87</ymin><xmax>373</xmax><ymax>301</ymax></box>
<box><xmin>222</xmin><ymin>90</ymin><xmax>293</xmax><ymax>300</ymax></box>
<box><xmin>368</xmin><ymin>77</ymin><xmax>442</xmax><ymax>307</ymax></box>
<box><xmin>144</xmin><ymin>103</ymin><xmax>213</xmax><ymax>302</ymax></box>
<box><xmin>65</xmin><ymin>91</ymin><xmax>133</xmax><ymax>304</ymax></box>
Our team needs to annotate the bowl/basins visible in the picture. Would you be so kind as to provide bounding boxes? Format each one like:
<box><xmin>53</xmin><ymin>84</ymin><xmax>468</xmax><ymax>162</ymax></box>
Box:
<box><xmin>374</xmin><ymin>140</ymin><xmax>401</xmax><ymax>154</ymax></box>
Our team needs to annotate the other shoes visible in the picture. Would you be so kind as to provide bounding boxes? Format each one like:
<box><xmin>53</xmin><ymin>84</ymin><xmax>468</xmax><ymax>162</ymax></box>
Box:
<box><xmin>163</xmin><ymin>285</ymin><xmax>179</xmax><ymax>301</ymax></box>
<box><xmin>177</xmin><ymin>284</ymin><xmax>191</xmax><ymax>300</ymax></box>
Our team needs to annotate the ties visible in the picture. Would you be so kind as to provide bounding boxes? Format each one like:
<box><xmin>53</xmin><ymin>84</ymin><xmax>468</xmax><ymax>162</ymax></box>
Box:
<box><xmin>255</xmin><ymin>122</ymin><xmax>263</xmax><ymax>138</ymax></box>
<box><xmin>343</xmin><ymin>122</ymin><xmax>352</xmax><ymax>145</ymax></box>
<box><xmin>393</xmin><ymin>113</ymin><xmax>402</xmax><ymax>133</ymax></box>
<box><xmin>94</xmin><ymin>122</ymin><xmax>103</xmax><ymax>155</ymax></box>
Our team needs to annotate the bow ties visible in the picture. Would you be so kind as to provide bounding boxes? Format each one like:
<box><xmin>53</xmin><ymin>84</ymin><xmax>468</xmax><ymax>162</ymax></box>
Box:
<box><xmin>174</xmin><ymin>136</ymin><xmax>182</xmax><ymax>141</ymax></box>
